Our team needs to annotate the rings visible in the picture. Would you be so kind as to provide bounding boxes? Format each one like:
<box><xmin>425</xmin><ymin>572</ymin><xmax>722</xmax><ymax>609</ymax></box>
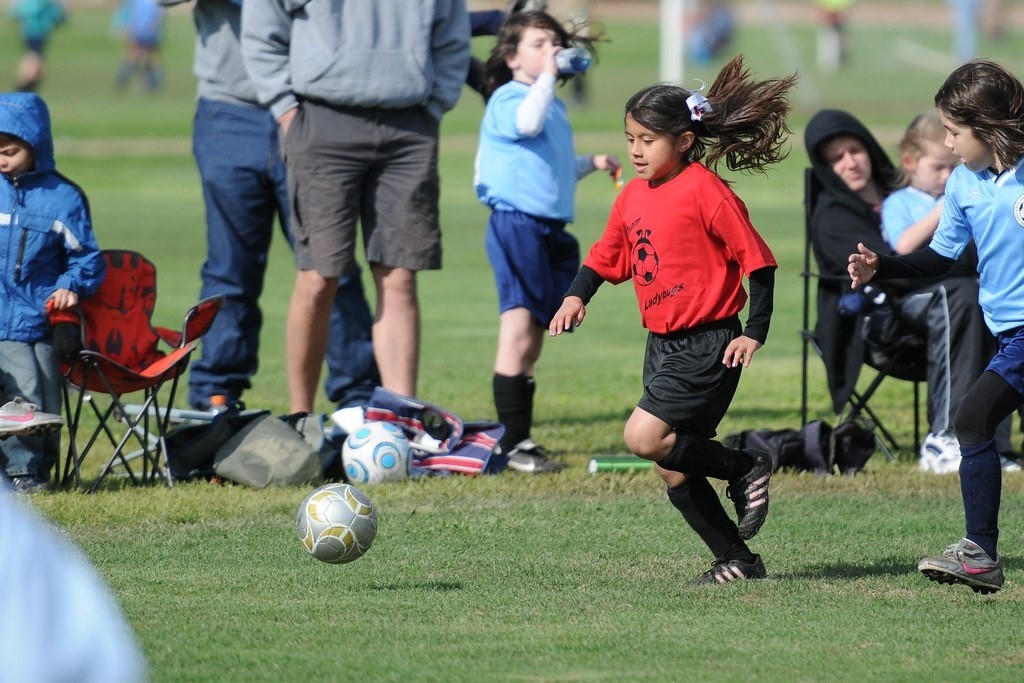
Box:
<box><xmin>855</xmin><ymin>270</ymin><xmax>859</xmax><ymax>276</ymax></box>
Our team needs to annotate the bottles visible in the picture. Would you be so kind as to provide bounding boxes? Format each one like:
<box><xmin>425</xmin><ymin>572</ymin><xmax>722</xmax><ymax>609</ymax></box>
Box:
<box><xmin>555</xmin><ymin>48</ymin><xmax>592</xmax><ymax>76</ymax></box>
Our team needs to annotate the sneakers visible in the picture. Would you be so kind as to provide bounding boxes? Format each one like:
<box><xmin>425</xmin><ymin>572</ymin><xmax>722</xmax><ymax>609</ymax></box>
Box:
<box><xmin>919</xmin><ymin>433</ymin><xmax>970</xmax><ymax>475</ymax></box>
<box><xmin>0</xmin><ymin>396</ymin><xmax>64</xmax><ymax>441</ymax></box>
<box><xmin>917</xmin><ymin>535</ymin><xmax>1004</xmax><ymax>597</ymax></box>
<box><xmin>500</xmin><ymin>438</ymin><xmax>568</xmax><ymax>473</ymax></box>
<box><xmin>684</xmin><ymin>552</ymin><xmax>768</xmax><ymax>591</ymax></box>
<box><xmin>1000</xmin><ymin>452</ymin><xmax>1022</xmax><ymax>474</ymax></box>
<box><xmin>725</xmin><ymin>448</ymin><xmax>774</xmax><ymax>540</ymax></box>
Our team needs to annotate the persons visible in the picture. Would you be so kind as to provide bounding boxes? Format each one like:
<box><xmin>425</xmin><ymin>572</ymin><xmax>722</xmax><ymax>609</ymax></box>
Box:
<box><xmin>0</xmin><ymin>91</ymin><xmax>107</xmax><ymax>497</ymax></box>
<box><xmin>111</xmin><ymin>0</ymin><xmax>167</xmax><ymax>91</ymax></box>
<box><xmin>805</xmin><ymin>109</ymin><xmax>1024</xmax><ymax>473</ymax></box>
<box><xmin>239</xmin><ymin>0</ymin><xmax>471</xmax><ymax>440</ymax></box>
<box><xmin>155</xmin><ymin>0</ymin><xmax>384</xmax><ymax>422</ymax></box>
<box><xmin>847</xmin><ymin>54</ymin><xmax>1024</xmax><ymax>594</ymax></box>
<box><xmin>547</xmin><ymin>54</ymin><xmax>795</xmax><ymax>585</ymax></box>
<box><xmin>473</xmin><ymin>10</ymin><xmax>619</xmax><ymax>472</ymax></box>
<box><xmin>10</xmin><ymin>0</ymin><xmax>65</xmax><ymax>93</ymax></box>
<box><xmin>872</xmin><ymin>109</ymin><xmax>962</xmax><ymax>258</ymax></box>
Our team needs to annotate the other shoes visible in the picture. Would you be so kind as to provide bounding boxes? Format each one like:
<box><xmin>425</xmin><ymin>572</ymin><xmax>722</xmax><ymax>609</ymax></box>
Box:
<box><xmin>12</xmin><ymin>477</ymin><xmax>51</xmax><ymax>497</ymax></box>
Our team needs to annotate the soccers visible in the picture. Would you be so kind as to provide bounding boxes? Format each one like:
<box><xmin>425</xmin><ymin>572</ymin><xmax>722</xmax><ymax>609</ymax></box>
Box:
<box><xmin>341</xmin><ymin>421</ymin><xmax>412</xmax><ymax>485</ymax></box>
<box><xmin>295</xmin><ymin>483</ymin><xmax>378</xmax><ymax>566</ymax></box>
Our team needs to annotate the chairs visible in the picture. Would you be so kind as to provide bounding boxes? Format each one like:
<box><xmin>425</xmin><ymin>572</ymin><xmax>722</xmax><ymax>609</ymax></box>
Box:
<box><xmin>795</xmin><ymin>164</ymin><xmax>931</xmax><ymax>465</ymax></box>
<box><xmin>44</xmin><ymin>245</ymin><xmax>224</xmax><ymax>492</ymax></box>
